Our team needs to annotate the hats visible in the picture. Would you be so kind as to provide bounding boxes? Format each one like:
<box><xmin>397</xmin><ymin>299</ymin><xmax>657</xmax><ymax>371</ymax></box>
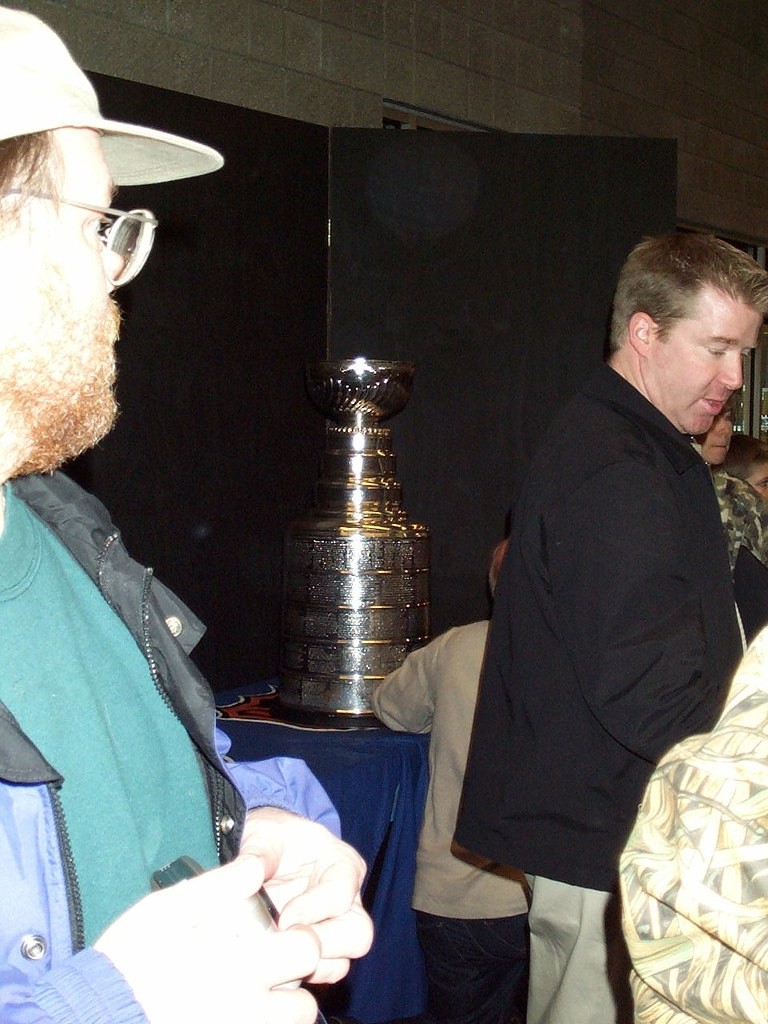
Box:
<box><xmin>0</xmin><ymin>6</ymin><xmax>225</xmax><ymax>187</ymax></box>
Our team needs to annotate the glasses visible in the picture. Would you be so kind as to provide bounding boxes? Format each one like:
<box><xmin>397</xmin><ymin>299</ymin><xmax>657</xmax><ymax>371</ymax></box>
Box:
<box><xmin>7</xmin><ymin>189</ymin><xmax>158</xmax><ymax>289</ymax></box>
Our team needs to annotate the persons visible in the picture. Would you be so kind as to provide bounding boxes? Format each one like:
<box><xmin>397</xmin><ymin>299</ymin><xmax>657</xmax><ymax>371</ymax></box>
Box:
<box><xmin>375</xmin><ymin>229</ymin><xmax>767</xmax><ymax>1024</ymax></box>
<box><xmin>0</xmin><ymin>3</ymin><xmax>375</xmax><ymax>1023</ymax></box>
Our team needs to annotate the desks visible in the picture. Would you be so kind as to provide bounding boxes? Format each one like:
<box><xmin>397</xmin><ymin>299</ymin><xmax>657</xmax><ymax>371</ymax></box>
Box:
<box><xmin>215</xmin><ymin>677</ymin><xmax>431</xmax><ymax>1024</ymax></box>
